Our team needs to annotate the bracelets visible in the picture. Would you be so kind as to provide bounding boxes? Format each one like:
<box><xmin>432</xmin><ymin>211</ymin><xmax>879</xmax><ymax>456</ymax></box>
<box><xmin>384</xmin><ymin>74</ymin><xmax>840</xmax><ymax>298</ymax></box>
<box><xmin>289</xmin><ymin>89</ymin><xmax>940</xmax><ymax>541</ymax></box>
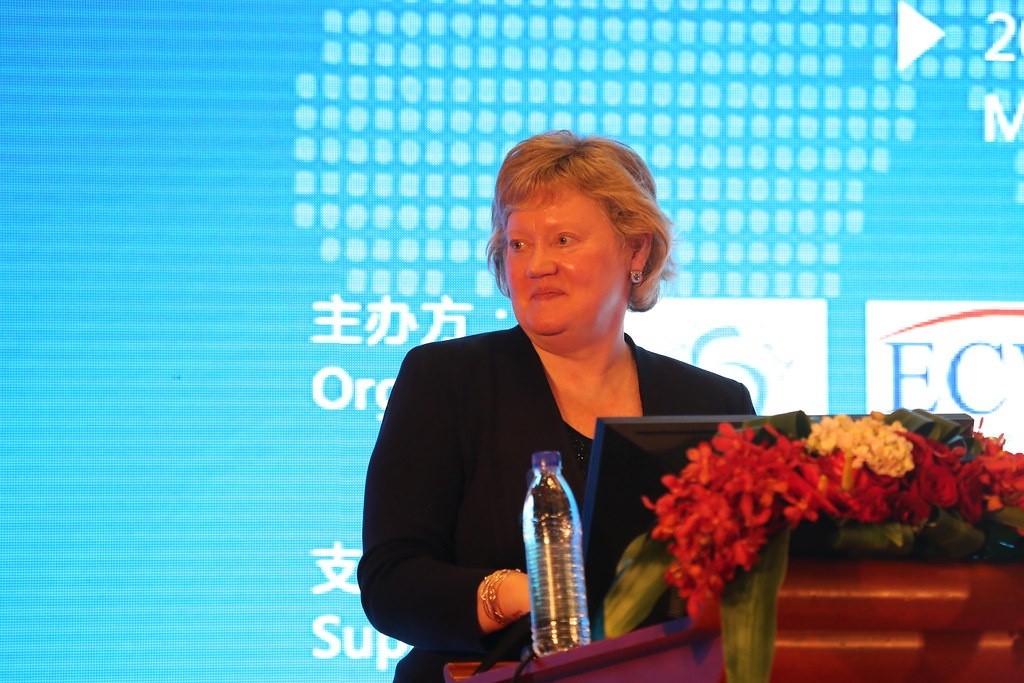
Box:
<box><xmin>482</xmin><ymin>568</ymin><xmax>522</xmax><ymax>623</ymax></box>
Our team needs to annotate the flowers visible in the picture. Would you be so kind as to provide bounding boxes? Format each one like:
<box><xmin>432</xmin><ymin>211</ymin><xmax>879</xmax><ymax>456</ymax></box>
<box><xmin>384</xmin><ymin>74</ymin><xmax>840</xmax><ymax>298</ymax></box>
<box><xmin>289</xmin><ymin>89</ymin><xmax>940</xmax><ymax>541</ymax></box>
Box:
<box><xmin>602</xmin><ymin>408</ymin><xmax>1024</xmax><ymax>683</ymax></box>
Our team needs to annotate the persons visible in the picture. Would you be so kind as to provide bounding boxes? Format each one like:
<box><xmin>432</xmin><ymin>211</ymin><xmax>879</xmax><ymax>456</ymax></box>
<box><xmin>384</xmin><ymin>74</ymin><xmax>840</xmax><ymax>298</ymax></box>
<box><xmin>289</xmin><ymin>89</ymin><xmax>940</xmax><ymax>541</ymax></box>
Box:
<box><xmin>358</xmin><ymin>132</ymin><xmax>756</xmax><ymax>683</ymax></box>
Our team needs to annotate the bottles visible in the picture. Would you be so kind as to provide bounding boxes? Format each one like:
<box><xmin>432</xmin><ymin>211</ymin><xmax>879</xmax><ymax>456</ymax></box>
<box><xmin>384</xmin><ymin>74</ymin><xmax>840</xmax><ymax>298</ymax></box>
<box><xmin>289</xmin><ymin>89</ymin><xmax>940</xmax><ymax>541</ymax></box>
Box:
<box><xmin>522</xmin><ymin>451</ymin><xmax>593</xmax><ymax>658</ymax></box>
